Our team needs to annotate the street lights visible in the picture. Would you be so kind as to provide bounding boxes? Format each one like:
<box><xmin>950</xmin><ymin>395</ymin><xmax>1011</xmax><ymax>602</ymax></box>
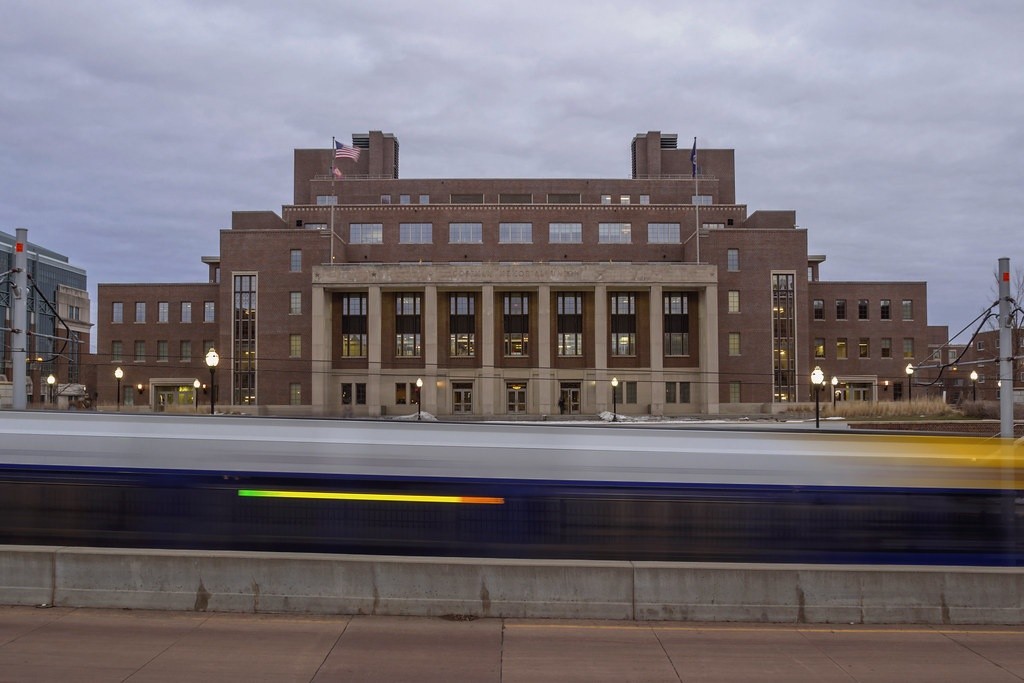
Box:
<box><xmin>904</xmin><ymin>363</ymin><xmax>916</xmax><ymax>406</ymax></box>
<box><xmin>811</xmin><ymin>365</ymin><xmax>824</xmax><ymax>428</ymax></box>
<box><xmin>115</xmin><ymin>367</ymin><xmax>124</xmax><ymax>412</ymax></box>
<box><xmin>47</xmin><ymin>374</ymin><xmax>57</xmax><ymax>404</ymax></box>
<box><xmin>970</xmin><ymin>370</ymin><xmax>978</xmax><ymax>402</ymax></box>
<box><xmin>205</xmin><ymin>347</ymin><xmax>220</xmax><ymax>415</ymax></box>
<box><xmin>194</xmin><ymin>378</ymin><xmax>201</xmax><ymax>412</ymax></box>
<box><xmin>416</xmin><ymin>376</ymin><xmax>424</xmax><ymax>421</ymax></box>
<box><xmin>831</xmin><ymin>376</ymin><xmax>839</xmax><ymax>412</ymax></box>
<box><xmin>609</xmin><ymin>375</ymin><xmax>621</xmax><ymax>422</ymax></box>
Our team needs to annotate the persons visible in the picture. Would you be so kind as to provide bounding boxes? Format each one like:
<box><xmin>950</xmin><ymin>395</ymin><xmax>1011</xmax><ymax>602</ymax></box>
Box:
<box><xmin>558</xmin><ymin>397</ymin><xmax>565</xmax><ymax>414</ymax></box>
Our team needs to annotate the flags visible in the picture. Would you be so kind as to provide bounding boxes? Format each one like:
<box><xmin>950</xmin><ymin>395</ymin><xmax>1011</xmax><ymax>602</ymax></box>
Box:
<box><xmin>690</xmin><ymin>140</ymin><xmax>697</xmax><ymax>177</ymax></box>
<box><xmin>335</xmin><ymin>140</ymin><xmax>361</xmax><ymax>163</ymax></box>
